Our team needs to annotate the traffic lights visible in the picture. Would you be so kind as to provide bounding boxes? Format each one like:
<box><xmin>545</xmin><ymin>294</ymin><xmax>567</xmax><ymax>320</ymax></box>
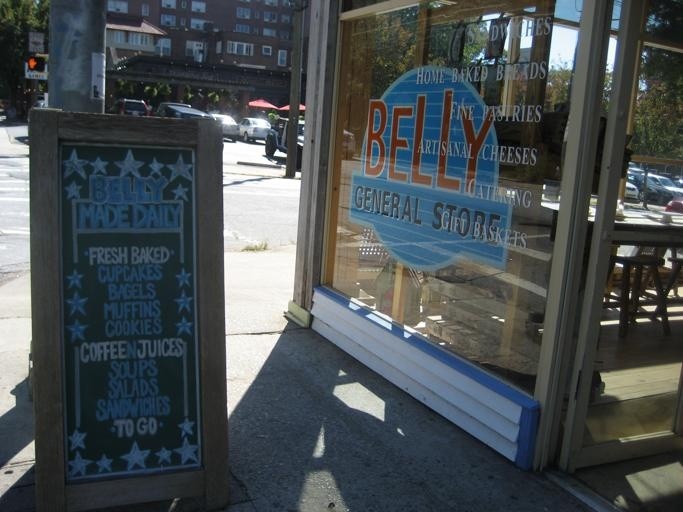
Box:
<box><xmin>27</xmin><ymin>56</ymin><xmax>44</xmax><ymax>70</ymax></box>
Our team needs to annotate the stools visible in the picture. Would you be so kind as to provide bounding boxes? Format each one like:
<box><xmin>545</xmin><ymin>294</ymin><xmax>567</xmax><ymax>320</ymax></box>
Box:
<box><xmin>602</xmin><ymin>255</ymin><xmax>683</xmax><ymax>338</ymax></box>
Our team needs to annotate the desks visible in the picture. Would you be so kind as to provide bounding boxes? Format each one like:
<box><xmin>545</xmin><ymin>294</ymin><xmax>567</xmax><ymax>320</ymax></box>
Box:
<box><xmin>541</xmin><ymin>200</ymin><xmax>683</xmax><ymax>351</ymax></box>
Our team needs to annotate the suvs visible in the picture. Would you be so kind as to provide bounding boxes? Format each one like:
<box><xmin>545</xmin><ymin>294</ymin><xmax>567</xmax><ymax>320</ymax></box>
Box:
<box><xmin>110</xmin><ymin>97</ymin><xmax>149</xmax><ymax>117</ymax></box>
<box><xmin>265</xmin><ymin>117</ymin><xmax>305</xmax><ymax>170</ymax></box>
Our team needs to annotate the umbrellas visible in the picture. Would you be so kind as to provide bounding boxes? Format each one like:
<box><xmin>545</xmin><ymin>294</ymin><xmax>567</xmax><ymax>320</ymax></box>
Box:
<box><xmin>279</xmin><ymin>102</ymin><xmax>305</xmax><ymax>111</ymax></box>
<box><xmin>247</xmin><ymin>97</ymin><xmax>278</xmax><ymax>111</ymax></box>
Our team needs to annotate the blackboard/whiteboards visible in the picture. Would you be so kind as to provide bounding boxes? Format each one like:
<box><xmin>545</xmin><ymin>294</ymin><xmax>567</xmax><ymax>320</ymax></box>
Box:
<box><xmin>28</xmin><ymin>107</ymin><xmax>230</xmax><ymax>512</ymax></box>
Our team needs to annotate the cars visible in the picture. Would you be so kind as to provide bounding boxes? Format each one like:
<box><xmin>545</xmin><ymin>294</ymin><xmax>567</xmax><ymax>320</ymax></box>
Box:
<box><xmin>623</xmin><ymin>162</ymin><xmax>682</xmax><ymax>215</ymax></box>
<box><xmin>157</xmin><ymin>102</ymin><xmax>211</xmax><ymax>117</ymax></box>
<box><xmin>209</xmin><ymin>114</ymin><xmax>241</xmax><ymax>143</ymax></box>
<box><xmin>238</xmin><ymin>117</ymin><xmax>272</xmax><ymax>143</ymax></box>
<box><xmin>341</xmin><ymin>129</ymin><xmax>356</xmax><ymax>162</ymax></box>
